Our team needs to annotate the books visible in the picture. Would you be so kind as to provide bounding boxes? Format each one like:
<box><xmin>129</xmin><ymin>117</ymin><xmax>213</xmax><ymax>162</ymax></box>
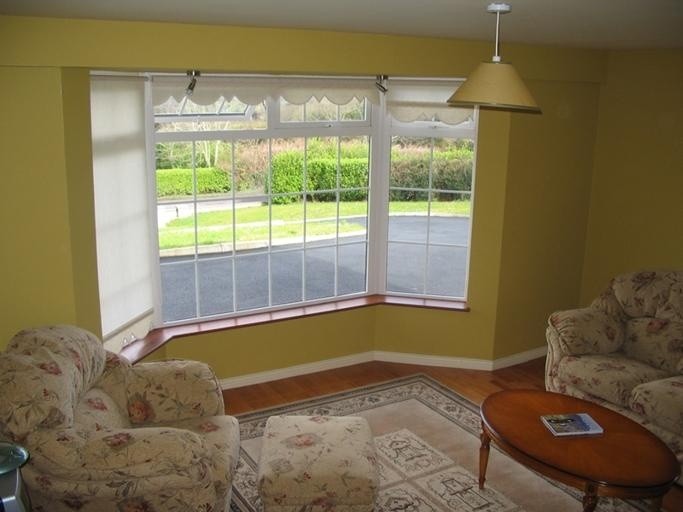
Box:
<box><xmin>539</xmin><ymin>412</ymin><xmax>603</xmax><ymax>435</ymax></box>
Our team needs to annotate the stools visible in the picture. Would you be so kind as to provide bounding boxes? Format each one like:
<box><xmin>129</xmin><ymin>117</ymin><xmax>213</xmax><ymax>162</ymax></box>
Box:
<box><xmin>257</xmin><ymin>416</ymin><xmax>378</xmax><ymax>512</ymax></box>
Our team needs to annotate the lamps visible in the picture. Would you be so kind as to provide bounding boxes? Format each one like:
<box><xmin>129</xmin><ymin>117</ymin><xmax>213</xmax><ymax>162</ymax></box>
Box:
<box><xmin>445</xmin><ymin>2</ymin><xmax>543</xmax><ymax>116</ymax></box>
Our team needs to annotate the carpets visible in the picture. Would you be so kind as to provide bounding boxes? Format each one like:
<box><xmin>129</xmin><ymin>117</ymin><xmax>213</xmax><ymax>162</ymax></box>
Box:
<box><xmin>231</xmin><ymin>372</ymin><xmax>670</xmax><ymax>512</ymax></box>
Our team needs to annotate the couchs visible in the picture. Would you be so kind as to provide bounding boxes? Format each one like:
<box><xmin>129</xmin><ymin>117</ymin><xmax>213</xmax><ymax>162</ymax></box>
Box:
<box><xmin>1</xmin><ymin>325</ymin><xmax>240</xmax><ymax>512</ymax></box>
<box><xmin>544</xmin><ymin>270</ymin><xmax>683</xmax><ymax>490</ymax></box>
